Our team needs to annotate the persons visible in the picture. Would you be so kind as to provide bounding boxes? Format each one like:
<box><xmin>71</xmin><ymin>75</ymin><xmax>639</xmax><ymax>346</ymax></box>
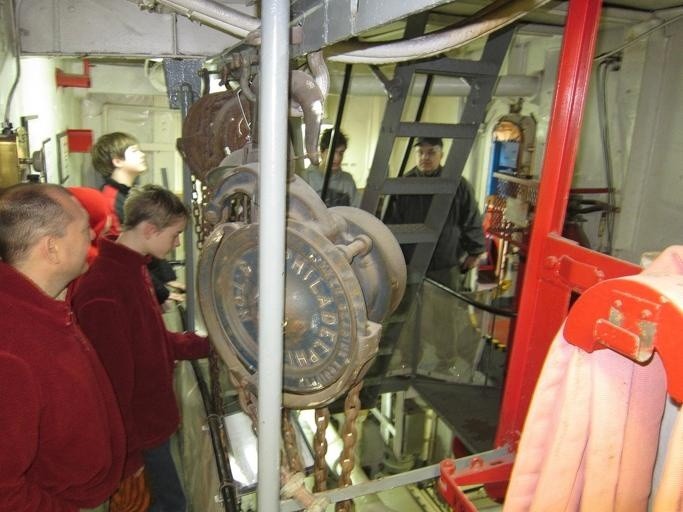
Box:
<box><xmin>308</xmin><ymin>129</ymin><xmax>358</xmax><ymax>208</ymax></box>
<box><xmin>90</xmin><ymin>133</ymin><xmax>186</xmax><ymax>313</ymax></box>
<box><xmin>382</xmin><ymin>136</ymin><xmax>487</xmax><ymax>379</ymax></box>
<box><xmin>71</xmin><ymin>184</ymin><xmax>210</xmax><ymax>512</ymax></box>
<box><xmin>0</xmin><ymin>183</ymin><xmax>126</xmax><ymax>512</ymax></box>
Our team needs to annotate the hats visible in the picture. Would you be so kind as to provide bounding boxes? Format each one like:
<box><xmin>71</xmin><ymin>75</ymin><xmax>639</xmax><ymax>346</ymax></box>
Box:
<box><xmin>413</xmin><ymin>135</ymin><xmax>443</xmax><ymax>146</ymax></box>
